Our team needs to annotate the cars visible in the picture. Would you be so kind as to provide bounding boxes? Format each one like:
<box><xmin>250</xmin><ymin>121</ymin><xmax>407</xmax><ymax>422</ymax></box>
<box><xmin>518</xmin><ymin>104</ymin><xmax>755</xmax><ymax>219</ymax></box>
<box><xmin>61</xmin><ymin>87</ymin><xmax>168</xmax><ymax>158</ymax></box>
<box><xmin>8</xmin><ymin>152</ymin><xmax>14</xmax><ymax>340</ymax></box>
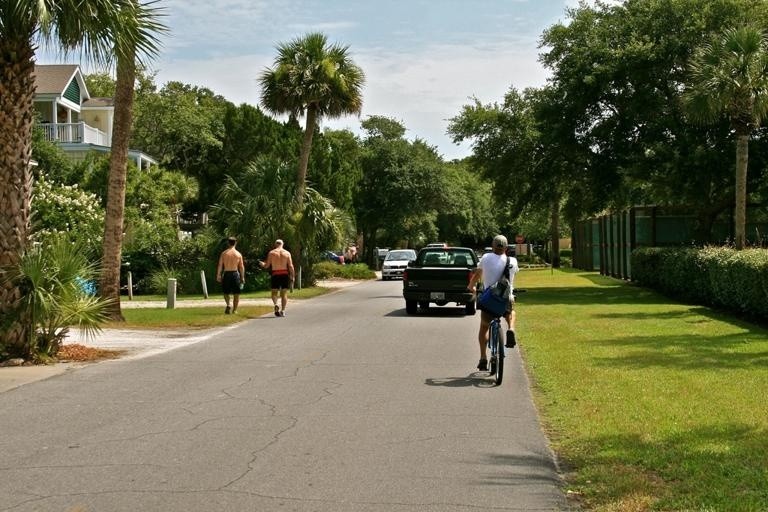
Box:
<box><xmin>381</xmin><ymin>249</ymin><xmax>419</xmax><ymax>279</ymax></box>
<box><xmin>319</xmin><ymin>250</ymin><xmax>346</xmax><ymax>266</ymax></box>
<box><xmin>481</xmin><ymin>247</ymin><xmax>493</xmax><ymax>256</ymax></box>
<box><xmin>505</xmin><ymin>245</ymin><xmax>516</xmax><ymax>255</ymax></box>
<box><xmin>74</xmin><ymin>273</ymin><xmax>97</xmax><ymax>299</ymax></box>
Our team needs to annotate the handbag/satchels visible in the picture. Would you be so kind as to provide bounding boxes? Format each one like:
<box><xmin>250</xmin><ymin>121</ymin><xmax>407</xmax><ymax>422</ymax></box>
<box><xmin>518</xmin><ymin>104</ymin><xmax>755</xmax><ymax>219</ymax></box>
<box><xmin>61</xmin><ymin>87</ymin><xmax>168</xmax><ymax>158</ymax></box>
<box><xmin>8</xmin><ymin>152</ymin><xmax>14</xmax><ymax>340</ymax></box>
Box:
<box><xmin>478</xmin><ymin>277</ymin><xmax>514</xmax><ymax>319</ymax></box>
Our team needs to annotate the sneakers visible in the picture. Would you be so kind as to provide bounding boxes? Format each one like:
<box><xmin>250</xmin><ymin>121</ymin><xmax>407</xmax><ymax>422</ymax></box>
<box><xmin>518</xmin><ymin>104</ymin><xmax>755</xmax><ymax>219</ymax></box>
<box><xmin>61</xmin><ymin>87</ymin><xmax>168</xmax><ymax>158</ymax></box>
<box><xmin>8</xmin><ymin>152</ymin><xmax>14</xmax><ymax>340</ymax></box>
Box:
<box><xmin>232</xmin><ymin>309</ymin><xmax>238</xmax><ymax>314</ymax></box>
<box><xmin>274</xmin><ymin>305</ymin><xmax>280</xmax><ymax>317</ymax></box>
<box><xmin>225</xmin><ymin>304</ymin><xmax>231</xmax><ymax>314</ymax></box>
<box><xmin>477</xmin><ymin>358</ymin><xmax>489</xmax><ymax>373</ymax></box>
<box><xmin>279</xmin><ymin>310</ymin><xmax>286</xmax><ymax>317</ymax></box>
<box><xmin>505</xmin><ymin>328</ymin><xmax>517</xmax><ymax>350</ymax></box>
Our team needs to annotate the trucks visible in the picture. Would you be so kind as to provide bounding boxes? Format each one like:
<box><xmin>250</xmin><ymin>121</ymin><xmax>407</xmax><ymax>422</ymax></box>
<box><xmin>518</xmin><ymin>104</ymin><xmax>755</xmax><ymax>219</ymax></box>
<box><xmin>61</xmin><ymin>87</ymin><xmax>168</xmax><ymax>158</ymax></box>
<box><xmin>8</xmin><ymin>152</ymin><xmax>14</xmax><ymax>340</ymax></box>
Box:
<box><xmin>423</xmin><ymin>243</ymin><xmax>452</xmax><ymax>264</ymax></box>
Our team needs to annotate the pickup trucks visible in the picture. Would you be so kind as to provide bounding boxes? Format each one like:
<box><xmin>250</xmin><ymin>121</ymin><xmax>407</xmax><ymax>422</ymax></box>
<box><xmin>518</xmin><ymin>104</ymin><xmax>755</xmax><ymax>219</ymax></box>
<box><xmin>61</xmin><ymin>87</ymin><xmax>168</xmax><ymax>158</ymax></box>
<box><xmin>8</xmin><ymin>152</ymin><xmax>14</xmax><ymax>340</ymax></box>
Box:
<box><xmin>402</xmin><ymin>246</ymin><xmax>480</xmax><ymax>314</ymax></box>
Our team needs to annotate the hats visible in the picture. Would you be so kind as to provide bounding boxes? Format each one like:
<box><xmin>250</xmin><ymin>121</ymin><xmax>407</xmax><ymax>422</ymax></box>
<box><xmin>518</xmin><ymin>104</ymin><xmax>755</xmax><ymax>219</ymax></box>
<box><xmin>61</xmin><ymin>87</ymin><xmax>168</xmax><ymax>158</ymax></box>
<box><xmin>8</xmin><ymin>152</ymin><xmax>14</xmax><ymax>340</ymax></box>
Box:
<box><xmin>492</xmin><ymin>234</ymin><xmax>509</xmax><ymax>252</ymax></box>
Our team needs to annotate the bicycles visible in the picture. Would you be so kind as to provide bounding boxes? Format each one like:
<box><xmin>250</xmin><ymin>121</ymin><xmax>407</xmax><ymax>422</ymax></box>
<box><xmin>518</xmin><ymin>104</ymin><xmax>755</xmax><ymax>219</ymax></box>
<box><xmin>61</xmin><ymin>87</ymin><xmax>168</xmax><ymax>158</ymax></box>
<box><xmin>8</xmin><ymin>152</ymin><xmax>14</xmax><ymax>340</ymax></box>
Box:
<box><xmin>465</xmin><ymin>288</ymin><xmax>528</xmax><ymax>385</ymax></box>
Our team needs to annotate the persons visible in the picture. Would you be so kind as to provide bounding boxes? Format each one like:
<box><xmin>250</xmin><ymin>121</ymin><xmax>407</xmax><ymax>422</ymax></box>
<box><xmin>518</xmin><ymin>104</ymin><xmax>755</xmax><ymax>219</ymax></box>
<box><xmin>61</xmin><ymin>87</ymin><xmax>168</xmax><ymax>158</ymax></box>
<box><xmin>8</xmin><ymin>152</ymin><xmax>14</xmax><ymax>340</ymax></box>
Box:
<box><xmin>258</xmin><ymin>240</ymin><xmax>295</xmax><ymax>317</ymax></box>
<box><xmin>337</xmin><ymin>243</ymin><xmax>360</xmax><ymax>266</ymax></box>
<box><xmin>466</xmin><ymin>234</ymin><xmax>516</xmax><ymax>369</ymax></box>
<box><xmin>216</xmin><ymin>237</ymin><xmax>245</xmax><ymax>314</ymax></box>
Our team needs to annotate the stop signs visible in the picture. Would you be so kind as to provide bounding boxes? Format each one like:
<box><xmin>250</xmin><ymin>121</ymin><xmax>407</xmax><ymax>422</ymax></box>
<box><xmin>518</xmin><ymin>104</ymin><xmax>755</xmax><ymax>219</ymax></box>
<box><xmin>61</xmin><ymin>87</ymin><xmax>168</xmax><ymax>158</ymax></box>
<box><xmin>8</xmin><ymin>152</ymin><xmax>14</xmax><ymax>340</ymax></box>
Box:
<box><xmin>514</xmin><ymin>235</ymin><xmax>525</xmax><ymax>244</ymax></box>
